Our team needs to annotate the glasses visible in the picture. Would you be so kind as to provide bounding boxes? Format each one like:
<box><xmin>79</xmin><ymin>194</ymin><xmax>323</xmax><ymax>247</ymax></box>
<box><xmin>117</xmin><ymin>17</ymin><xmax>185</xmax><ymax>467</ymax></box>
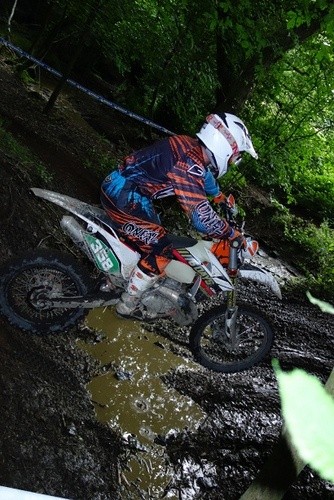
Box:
<box><xmin>230</xmin><ymin>152</ymin><xmax>242</xmax><ymax>169</ymax></box>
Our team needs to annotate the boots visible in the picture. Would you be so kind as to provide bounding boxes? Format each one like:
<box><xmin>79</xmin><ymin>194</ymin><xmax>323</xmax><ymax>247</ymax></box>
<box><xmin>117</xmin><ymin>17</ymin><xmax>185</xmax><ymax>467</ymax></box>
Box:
<box><xmin>116</xmin><ymin>264</ymin><xmax>159</xmax><ymax>323</ymax></box>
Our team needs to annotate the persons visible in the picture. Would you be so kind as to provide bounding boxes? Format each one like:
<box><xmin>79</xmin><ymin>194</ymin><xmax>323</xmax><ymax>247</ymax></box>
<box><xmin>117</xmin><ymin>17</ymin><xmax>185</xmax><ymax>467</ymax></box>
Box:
<box><xmin>100</xmin><ymin>111</ymin><xmax>259</xmax><ymax>322</ymax></box>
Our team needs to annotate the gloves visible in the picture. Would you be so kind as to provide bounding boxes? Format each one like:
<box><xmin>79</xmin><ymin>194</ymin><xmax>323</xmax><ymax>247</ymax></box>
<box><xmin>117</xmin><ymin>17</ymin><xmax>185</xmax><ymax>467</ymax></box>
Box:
<box><xmin>213</xmin><ymin>191</ymin><xmax>227</xmax><ymax>208</ymax></box>
<box><xmin>227</xmin><ymin>228</ymin><xmax>247</xmax><ymax>253</ymax></box>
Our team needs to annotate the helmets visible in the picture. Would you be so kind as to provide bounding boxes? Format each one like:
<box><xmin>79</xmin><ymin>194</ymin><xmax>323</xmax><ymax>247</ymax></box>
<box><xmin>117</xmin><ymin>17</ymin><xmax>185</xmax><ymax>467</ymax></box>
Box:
<box><xmin>196</xmin><ymin>112</ymin><xmax>258</xmax><ymax>179</ymax></box>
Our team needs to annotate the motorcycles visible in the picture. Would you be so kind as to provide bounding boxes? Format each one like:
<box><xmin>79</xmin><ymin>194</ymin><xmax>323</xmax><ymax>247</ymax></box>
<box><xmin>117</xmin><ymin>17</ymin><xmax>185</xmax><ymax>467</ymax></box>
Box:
<box><xmin>0</xmin><ymin>186</ymin><xmax>282</xmax><ymax>373</ymax></box>
